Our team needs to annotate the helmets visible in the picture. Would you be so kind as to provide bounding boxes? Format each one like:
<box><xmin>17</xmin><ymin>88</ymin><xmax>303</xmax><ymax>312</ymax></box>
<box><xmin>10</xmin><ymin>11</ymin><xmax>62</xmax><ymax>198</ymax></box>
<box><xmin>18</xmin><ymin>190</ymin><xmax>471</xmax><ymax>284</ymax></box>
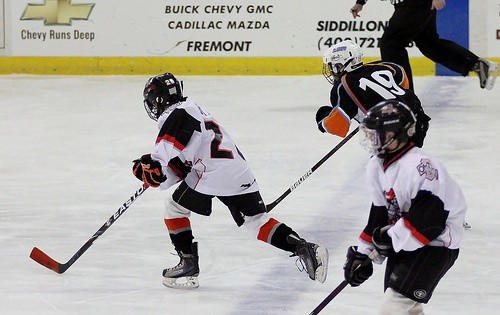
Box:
<box><xmin>358</xmin><ymin>99</ymin><xmax>417</xmax><ymax>159</ymax></box>
<box><xmin>321</xmin><ymin>40</ymin><xmax>364</xmax><ymax>84</ymax></box>
<box><xmin>143</xmin><ymin>71</ymin><xmax>183</xmax><ymax>122</ymax></box>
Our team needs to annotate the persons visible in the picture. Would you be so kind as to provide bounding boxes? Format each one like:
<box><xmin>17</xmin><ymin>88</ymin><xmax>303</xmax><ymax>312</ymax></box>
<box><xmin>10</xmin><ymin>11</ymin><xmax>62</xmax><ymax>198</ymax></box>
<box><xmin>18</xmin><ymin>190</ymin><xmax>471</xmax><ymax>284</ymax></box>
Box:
<box><xmin>338</xmin><ymin>100</ymin><xmax>467</xmax><ymax>315</ymax></box>
<box><xmin>350</xmin><ymin>0</ymin><xmax>498</xmax><ymax>95</ymax></box>
<box><xmin>316</xmin><ymin>41</ymin><xmax>432</xmax><ymax>153</ymax></box>
<box><xmin>126</xmin><ymin>72</ymin><xmax>330</xmax><ymax>290</ymax></box>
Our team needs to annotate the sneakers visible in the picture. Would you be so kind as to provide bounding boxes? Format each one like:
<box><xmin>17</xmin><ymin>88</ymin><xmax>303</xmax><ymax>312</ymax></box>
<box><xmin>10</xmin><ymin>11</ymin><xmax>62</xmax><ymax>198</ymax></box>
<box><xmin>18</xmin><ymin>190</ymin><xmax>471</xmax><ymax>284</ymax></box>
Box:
<box><xmin>162</xmin><ymin>252</ymin><xmax>200</xmax><ymax>288</ymax></box>
<box><xmin>473</xmin><ymin>58</ymin><xmax>498</xmax><ymax>90</ymax></box>
<box><xmin>287</xmin><ymin>233</ymin><xmax>329</xmax><ymax>282</ymax></box>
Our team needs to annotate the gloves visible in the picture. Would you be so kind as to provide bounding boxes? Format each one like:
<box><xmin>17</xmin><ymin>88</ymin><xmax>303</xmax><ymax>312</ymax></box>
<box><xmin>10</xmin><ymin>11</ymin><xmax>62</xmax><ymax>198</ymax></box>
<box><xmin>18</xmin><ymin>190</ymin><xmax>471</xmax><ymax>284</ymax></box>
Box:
<box><xmin>132</xmin><ymin>153</ymin><xmax>152</xmax><ymax>182</ymax></box>
<box><xmin>371</xmin><ymin>223</ymin><xmax>396</xmax><ymax>257</ymax></box>
<box><xmin>342</xmin><ymin>245</ymin><xmax>373</xmax><ymax>287</ymax></box>
<box><xmin>140</xmin><ymin>160</ymin><xmax>167</xmax><ymax>188</ymax></box>
<box><xmin>316</xmin><ymin>106</ymin><xmax>334</xmax><ymax>133</ymax></box>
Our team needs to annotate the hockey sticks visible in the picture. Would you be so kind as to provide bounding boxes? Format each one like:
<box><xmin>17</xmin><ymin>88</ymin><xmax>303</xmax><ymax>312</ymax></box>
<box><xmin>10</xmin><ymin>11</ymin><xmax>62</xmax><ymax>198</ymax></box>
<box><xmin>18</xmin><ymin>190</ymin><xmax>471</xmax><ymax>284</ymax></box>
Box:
<box><xmin>308</xmin><ymin>248</ymin><xmax>378</xmax><ymax>315</ymax></box>
<box><xmin>29</xmin><ymin>180</ymin><xmax>148</xmax><ymax>276</ymax></box>
<box><xmin>263</xmin><ymin>125</ymin><xmax>360</xmax><ymax>214</ymax></box>
